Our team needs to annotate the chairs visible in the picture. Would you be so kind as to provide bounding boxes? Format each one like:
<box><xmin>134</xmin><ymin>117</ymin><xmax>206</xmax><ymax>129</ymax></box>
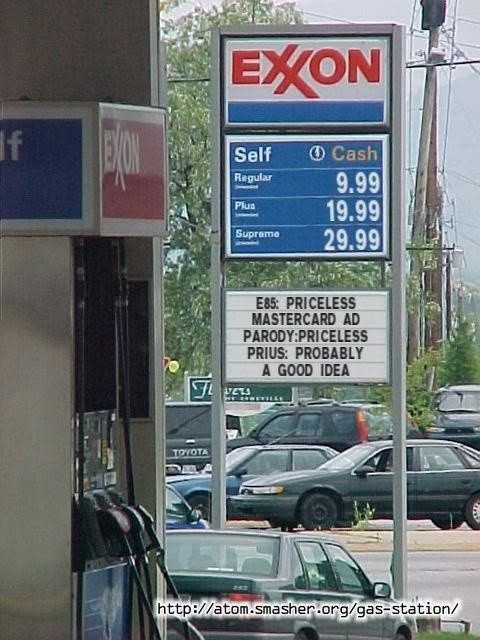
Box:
<box><xmin>187</xmin><ymin>553</ymin><xmax>211</xmax><ymax>571</ymax></box>
<box><xmin>241</xmin><ymin>556</ymin><xmax>276</xmax><ymax>576</ymax></box>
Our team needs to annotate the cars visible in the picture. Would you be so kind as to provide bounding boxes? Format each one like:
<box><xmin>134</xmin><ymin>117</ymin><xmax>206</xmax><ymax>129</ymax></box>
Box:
<box><xmin>160</xmin><ymin>528</ymin><xmax>418</xmax><ymax>640</ymax></box>
